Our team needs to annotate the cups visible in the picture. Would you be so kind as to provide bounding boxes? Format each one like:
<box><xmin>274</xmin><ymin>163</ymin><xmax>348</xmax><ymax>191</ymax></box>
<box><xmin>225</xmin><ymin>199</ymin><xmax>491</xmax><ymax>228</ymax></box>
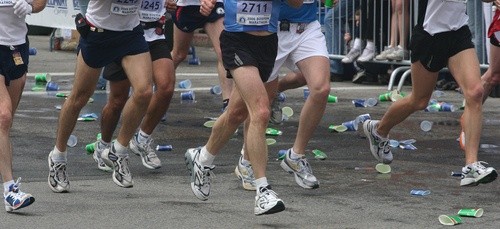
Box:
<box><xmin>324</xmin><ymin>0</ymin><xmax>334</xmax><ymax>9</ymax></box>
<box><xmin>438</xmin><ymin>215</ymin><xmax>462</xmax><ymax>225</ymax></box>
<box><xmin>29</xmin><ymin>47</ymin><xmax>464</xmax><ymax>196</ymax></box>
<box><xmin>458</xmin><ymin>208</ymin><xmax>483</xmax><ymax>217</ymax></box>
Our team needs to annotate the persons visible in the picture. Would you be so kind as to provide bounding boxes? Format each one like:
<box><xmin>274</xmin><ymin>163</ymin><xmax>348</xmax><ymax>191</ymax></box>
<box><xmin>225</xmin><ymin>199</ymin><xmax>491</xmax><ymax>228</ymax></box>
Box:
<box><xmin>363</xmin><ymin>0</ymin><xmax>497</xmax><ymax>187</ymax></box>
<box><xmin>459</xmin><ymin>0</ymin><xmax>500</xmax><ymax>151</ymax></box>
<box><xmin>0</xmin><ymin>0</ymin><xmax>47</xmax><ymax>212</ymax></box>
<box><xmin>342</xmin><ymin>1</ymin><xmax>409</xmax><ymax>84</ymax></box>
<box><xmin>93</xmin><ymin>0</ymin><xmax>178</xmax><ymax>173</ymax></box>
<box><xmin>234</xmin><ymin>0</ymin><xmax>331</xmax><ymax>190</ymax></box>
<box><xmin>266</xmin><ymin>52</ymin><xmax>308</xmax><ymax>124</ymax></box>
<box><xmin>170</xmin><ymin>0</ymin><xmax>239</xmax><ymax>136</ymax></box>
<box><xmin>186</xmin><ymin>1</ymin><xmax>303</xmax><ymax>215</ymax></box>
<box><xmin>48</xmin><ymin>0</ymin><xmax>153</xmax><ymax>193</ymax></box>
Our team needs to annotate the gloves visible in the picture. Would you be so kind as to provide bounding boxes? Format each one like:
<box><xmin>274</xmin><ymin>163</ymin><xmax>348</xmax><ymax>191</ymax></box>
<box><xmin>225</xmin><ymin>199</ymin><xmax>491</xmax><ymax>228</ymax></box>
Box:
<box><xmin>11</xmin><ymin>0</ymin><xmax>34</xmax><ymax>20</ymax></box>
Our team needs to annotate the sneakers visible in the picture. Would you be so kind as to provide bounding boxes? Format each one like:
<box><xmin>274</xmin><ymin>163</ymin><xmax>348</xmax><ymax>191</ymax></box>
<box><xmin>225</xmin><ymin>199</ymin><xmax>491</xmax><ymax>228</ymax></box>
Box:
<box><xmin>3</xmin><ymin>176</ymin><xmax>36</xmax><ymax>213</ymax></box>
<box><xmin>128</xmin><ymin>129</ymin><xmax>163</xmax><ymax>170</ymax></box>
<box><xmin>459</xmin><ymin>160</ymin><xmax>498</xmax><ymax>187</ymax></box>
<box><xmin>356</xmin><ymin>48</ymin><xmax>378</xmax><ymax>63</ymax></box>
<box><xmin>100</xmin><ymin>139</ymin><xmax>135</xmax><ymax>188</ymax></box>
<box><xmin>279</xmin><ymin>148</ymin><xmax>320</xmax><ymax>190</ymax></box>
<box><xmin>46</xmin><ymin>150</ymin><xmax>71</xmax><ymax>194</ymax></box>
<box><xmin>183</xmin><ymin>146</ymin><xmax>215</xmax><ymax>202</ymax></box>
<box><xmin>92</xmin><ymin>141</ymin><xmax>113</xmax><ymax>172</ymax></box>
<box><xmin>362</xmin><ymin>119</ymin><xmax>394</xmax><ymax>165</ymax></box>
<box><xmin>234</xmin><ymin>155</ymin><xmax>259</xmax><ymax>192</ymax></box>
<box><xmin>252</xmin><ymin>185</ymin><xmax>286</xmax><ymax>216</ymax></box>
<box><xmin>341</xmin><ymin>47</ymin><xmax>361</xmax><ymax>64</ymax></box>
<box><xmin>375</xmin><ymin>45</ymin><xmax>405</xmax><ymax>61</ymax></box>
